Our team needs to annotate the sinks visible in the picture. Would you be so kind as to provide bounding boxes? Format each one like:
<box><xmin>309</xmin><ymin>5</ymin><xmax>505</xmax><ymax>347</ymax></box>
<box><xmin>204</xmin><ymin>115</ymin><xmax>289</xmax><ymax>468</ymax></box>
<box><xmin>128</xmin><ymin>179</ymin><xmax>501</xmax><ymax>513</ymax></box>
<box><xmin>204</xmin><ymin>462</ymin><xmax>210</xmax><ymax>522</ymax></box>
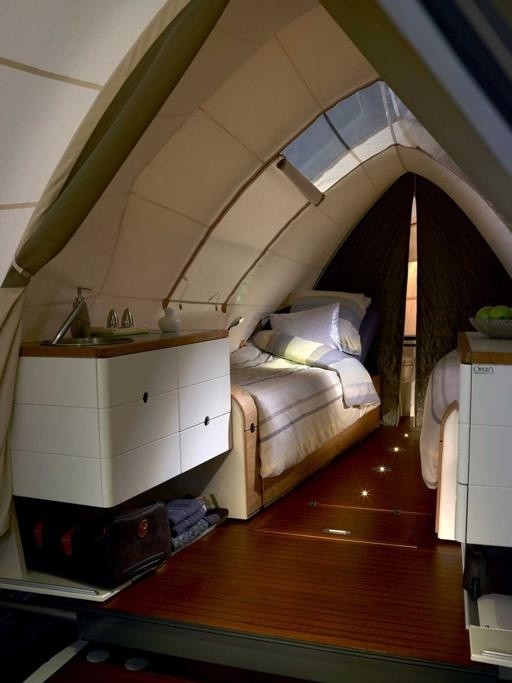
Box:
<box><xmin>40</xmin><ymin>337</ymin><xmax>134</xmax><ymax>346</ymax></box>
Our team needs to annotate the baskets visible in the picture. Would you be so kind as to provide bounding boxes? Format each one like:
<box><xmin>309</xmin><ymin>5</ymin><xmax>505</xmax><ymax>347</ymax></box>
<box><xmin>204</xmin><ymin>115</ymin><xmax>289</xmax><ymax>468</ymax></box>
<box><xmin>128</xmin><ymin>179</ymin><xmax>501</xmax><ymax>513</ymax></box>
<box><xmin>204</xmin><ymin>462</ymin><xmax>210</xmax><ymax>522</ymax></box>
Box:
<box><xmin>469</xmin><ymin>318</ymin><xmax>512</xmax><ymax>338</ymax></box>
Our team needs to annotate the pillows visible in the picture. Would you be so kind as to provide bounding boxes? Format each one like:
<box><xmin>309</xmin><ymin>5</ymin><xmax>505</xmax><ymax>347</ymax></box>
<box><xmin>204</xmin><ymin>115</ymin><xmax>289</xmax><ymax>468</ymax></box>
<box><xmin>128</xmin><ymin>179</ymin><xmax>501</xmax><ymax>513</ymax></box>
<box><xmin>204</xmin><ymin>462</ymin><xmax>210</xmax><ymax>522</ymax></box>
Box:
<box><xmin>269</xmin><ymin>290</ymin><xmax>372</xmax><ymax>357</ymax></box>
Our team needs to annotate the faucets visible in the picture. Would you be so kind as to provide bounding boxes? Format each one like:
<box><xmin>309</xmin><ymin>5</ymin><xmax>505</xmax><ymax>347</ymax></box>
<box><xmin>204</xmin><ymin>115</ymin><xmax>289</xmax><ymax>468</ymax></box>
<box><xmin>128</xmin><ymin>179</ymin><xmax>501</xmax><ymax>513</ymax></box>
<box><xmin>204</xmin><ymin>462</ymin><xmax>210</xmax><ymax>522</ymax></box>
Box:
<box><xmin>122</xmin><ymin>309</ymin><xmax>134</xmax><ymax>328</ymax></box>
<box><xmin>50</xmin><ymin>296</ymin><xmax>86</xmax><ymax>344</ymax></box>
<box><xmin>105</xmin><ymin>309</ymin><xmax>120</xmax><ymax>329</ymax></box>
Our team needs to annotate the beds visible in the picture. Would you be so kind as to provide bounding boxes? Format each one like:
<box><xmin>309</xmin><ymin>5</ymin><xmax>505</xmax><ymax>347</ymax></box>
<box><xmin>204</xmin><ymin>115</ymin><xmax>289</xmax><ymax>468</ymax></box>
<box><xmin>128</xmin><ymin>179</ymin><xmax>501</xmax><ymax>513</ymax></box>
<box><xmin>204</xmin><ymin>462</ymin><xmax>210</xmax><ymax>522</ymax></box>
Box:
<box><xmin>174</xmin><ymin>330</ymin><xmax>382</xmax><ymax>524</ymax></box>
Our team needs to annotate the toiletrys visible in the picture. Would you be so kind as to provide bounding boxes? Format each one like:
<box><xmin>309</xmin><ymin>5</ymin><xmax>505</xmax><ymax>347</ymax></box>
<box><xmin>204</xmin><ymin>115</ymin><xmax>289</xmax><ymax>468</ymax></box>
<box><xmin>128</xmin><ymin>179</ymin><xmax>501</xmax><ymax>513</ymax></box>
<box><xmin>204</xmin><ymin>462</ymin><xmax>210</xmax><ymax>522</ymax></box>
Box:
<box><xmin>70</xmin><ymin>286</ymin><xmax>93</xmax><ymax>339</ymax></box>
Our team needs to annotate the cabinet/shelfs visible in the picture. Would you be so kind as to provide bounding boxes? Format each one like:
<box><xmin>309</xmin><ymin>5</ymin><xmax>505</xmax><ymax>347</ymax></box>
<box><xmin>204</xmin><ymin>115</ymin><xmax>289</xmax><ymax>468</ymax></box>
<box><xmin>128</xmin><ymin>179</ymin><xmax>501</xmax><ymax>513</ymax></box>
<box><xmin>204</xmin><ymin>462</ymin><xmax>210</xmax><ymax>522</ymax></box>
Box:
<box><xmin>9</xmin><ymin>338</ymin><xmax>234</xmax><ymax>509</ymax></box>
